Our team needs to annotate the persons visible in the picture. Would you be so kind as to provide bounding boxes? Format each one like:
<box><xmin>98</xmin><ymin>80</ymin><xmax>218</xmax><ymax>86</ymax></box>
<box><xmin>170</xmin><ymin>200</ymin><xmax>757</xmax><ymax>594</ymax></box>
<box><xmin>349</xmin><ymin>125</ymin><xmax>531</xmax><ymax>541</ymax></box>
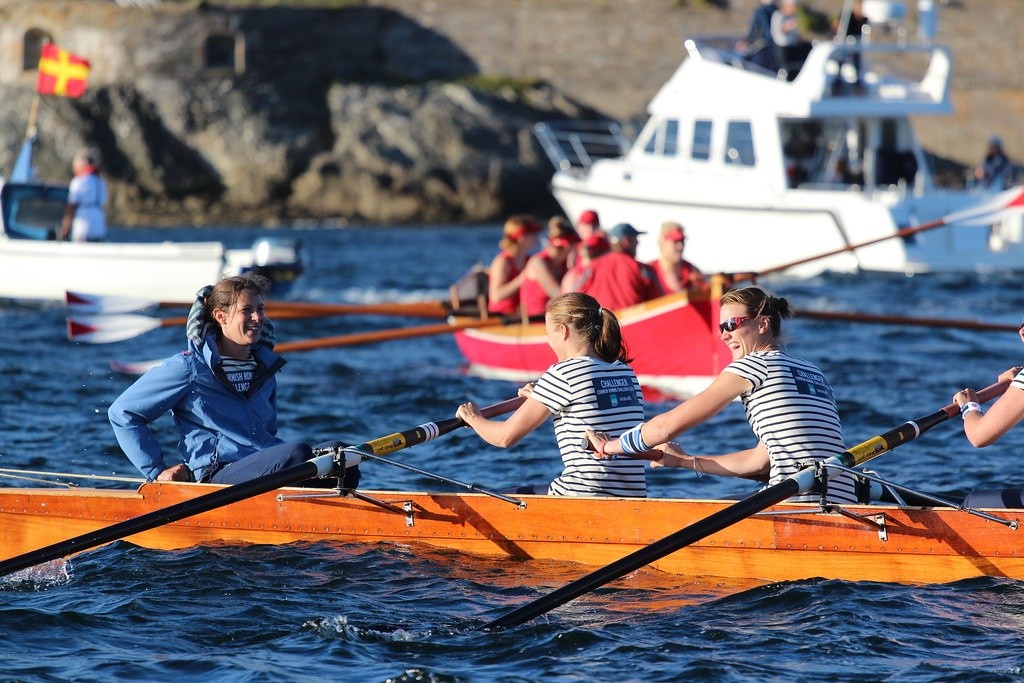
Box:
<box><xmin>952</xmin><ymin>327</ymin><xmax>1024</xmax><ymax>509</ymax></box>
<box><xmin>455</xmin><ymin>293</ymin><xmax>647</xmax><ymax>498</ymax></box>
<box><xmin>586</xmin><ymin>287</ymin><xmax>858</xmax><ymax>504</ymax></box>
<box><xmin>737</xmin><ymin>0</ymin><xmax>867</xmax><ymax>78</ymax></box>
<box><xmin>488</xmin><ymin>211</ymin><xmax>705</xmax><ymax>322</ymax></box>
<box><xmin>975</xmin><ymin>136</ymin><xmax>1010</xmax><ymax>192</ymax></box>
<box><xmin>61</xmin><ymin>154</ymin><xmax>107</xmax><ymax>242</ymax></box>
<box><xmin>108</xmin><ymin>276</ymin><xmax>360</xmax><ymax>489</ymax></box>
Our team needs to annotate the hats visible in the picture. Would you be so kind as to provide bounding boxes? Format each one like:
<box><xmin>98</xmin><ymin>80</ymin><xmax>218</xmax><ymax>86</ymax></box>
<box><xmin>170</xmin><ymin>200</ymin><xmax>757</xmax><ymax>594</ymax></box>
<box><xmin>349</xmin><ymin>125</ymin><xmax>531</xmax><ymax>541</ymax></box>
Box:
<box><xmin>508</xmin><ymin>221</ymin><xmax>544</xmax><ymax>240</ymax></box>
<box><xmin>661</xmin><ymin>222</ymin><xmax>684</xmax><ymax>241</ymax></box>
<box><xmin>580</xmin><ymin>211</ymin><xmax>599</xmax><ymax>225</ymax></box>
<box><xmin>607</xmin><ymin>224</ymin><xmax>646</xmax><ymax>238</ymax></box>
<box><xmin>529</xmin><ymin>230</ymin><xmax>530</xmax><ymax>231</ymax></box>
<box><xmin>549</xmin><ymin>234</ymin><xmax>573</xmax><ymax>246</ymax></box>
<box><xmin>578</xmin><ymin>235</ymin><xmax>608</xmax><ymax>255</ymax></box>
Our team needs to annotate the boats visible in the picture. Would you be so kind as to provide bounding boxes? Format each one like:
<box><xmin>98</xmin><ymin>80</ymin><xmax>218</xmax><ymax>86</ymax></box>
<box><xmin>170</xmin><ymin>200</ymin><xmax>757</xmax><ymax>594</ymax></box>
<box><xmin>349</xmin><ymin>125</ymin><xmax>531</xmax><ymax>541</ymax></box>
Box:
<box><xmin>0</xmin><ymin>124</ymin><xmax>303</xmax><ymax>310</ymax></box>
<box><xmin>531</xmin><ymin>0</ymin><xmax>1024</xmax><ymax>278</ymax></box>
<box><xmin>447</xmin><ymin>261</ymin><xmax>744</xmax><ymax>403</ymax></box>
<box><xmin>0</xmin><ymin>468</ymin><xmax>1024</xmax><ymax>586</ymax></box>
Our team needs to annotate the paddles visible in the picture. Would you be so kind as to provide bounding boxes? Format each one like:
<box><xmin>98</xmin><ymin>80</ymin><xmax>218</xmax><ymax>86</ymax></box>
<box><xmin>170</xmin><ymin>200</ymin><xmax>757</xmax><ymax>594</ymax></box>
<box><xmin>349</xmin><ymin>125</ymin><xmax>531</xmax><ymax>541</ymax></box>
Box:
<box><xmin>756</xmin><ymin>185</ymin><xmax>1023</xmax><ymax>277</ymax></box>
<box><xmin>580</xmin><ymin>438</ymin><xmax>1013</xmax><ymax>506</ymax></box>
<box><xmin>109</xmin><ymin>312</ymin><xmax>548</xmax><ymax>375</ymax></box>
<box><xmin>64</xmin><ymin>290</ymin><xmax>482</xmax><ymax>345</ymax></box>
<box><xmin>476</xmin><ymin>376</ymin><xmax>1013</xmax><ymax>634</ymax></box>
<box><xmin>790</xmin><ymin>308</ymin><xmax>1024</xmax><ymax>333</ymax></box>
<box><xmin>0</xmin><ymin>395</ymin><xmax>525</xmax><ymax>580</ymax></box>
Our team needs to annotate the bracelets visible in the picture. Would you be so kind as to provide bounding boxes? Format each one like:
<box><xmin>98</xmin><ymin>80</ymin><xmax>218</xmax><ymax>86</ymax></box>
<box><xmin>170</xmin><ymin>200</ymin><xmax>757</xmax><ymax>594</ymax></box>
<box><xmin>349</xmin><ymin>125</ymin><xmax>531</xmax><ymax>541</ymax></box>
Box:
<box><xmin>961</xmin><ymin>402</ymin><xmax>983</xmax><ymax>419</ymax></box>
<box><xmin>600</xmin><ymin>441</ymin><xmax>608</xmax><ymax>455</ymax></box>
<box><xmin>694</xmin><ymin>456</ymin><xmax>703</xmax><ymax>478</ymax></box>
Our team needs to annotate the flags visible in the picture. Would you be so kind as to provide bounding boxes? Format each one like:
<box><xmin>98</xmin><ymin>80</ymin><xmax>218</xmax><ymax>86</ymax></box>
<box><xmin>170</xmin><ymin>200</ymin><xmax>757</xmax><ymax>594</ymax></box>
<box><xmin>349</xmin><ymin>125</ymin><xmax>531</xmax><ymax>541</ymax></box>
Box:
<box><xmin>37</xmin><ymin>43</ymin><xmax>92</xmax><ymax>97</ymax></box>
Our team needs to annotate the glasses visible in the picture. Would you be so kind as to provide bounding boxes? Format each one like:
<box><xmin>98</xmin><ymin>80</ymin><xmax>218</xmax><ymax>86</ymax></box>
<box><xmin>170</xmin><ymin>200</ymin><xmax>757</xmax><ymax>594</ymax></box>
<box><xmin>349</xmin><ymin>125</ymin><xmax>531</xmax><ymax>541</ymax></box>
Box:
<box><xmin>719</xmin><ymin>315</ymin><xmax>771</xmax><ymax>334</ymax></box>
